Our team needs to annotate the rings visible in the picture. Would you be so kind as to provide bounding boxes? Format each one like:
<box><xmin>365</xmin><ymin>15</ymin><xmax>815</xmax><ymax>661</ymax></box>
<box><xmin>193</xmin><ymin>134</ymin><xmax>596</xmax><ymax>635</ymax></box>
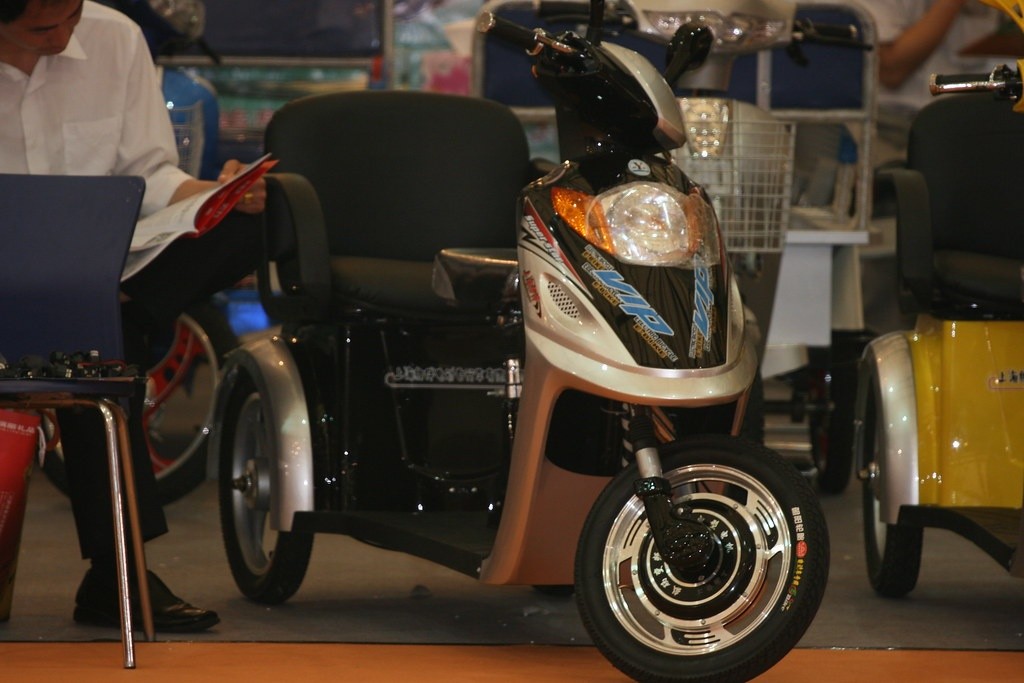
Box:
<box><xmin>244</xmin><ymin>193</ymin><xmax>253</xmax><ymax>205</ymax></box>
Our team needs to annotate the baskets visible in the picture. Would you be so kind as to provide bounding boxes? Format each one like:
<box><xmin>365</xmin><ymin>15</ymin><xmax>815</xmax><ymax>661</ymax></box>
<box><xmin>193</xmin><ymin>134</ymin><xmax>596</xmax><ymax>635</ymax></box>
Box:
<box><xmin>666</xmin><ymin>116</ymin><xmax>797</xmax><ymax>254</ymax></box>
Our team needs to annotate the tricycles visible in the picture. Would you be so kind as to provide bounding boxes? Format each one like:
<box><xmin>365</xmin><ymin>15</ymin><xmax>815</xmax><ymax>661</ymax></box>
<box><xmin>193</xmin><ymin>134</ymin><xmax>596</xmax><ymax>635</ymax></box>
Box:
<box><xmin>466</xmin><ymin>0</ymin><xmax>879</xmax><ymax>499</ymax></box>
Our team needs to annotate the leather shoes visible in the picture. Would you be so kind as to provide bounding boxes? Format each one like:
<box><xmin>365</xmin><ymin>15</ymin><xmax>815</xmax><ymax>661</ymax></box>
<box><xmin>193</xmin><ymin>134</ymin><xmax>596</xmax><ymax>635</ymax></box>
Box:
<box><xmin>73</xmin><ymin>567</ymin><xmax>220</xmax><ymax>633</ymax></box>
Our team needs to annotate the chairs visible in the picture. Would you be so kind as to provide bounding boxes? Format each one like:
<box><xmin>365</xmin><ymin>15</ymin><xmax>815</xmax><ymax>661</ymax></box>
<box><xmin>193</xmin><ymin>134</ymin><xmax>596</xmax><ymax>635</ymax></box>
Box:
<box><xmin>262</xmin><ymin>89</ymin><xmax>557</xmax><ymax>320</ymax></box>
<box><xmin>876</xmin><ymin>89</ymin><xmax>1024</xmax><ymax>319</ymax></box>
<box><xmin>0</xmin><ymin>174</ymin><xmax>156</xmax><ymax>670</ymax></box>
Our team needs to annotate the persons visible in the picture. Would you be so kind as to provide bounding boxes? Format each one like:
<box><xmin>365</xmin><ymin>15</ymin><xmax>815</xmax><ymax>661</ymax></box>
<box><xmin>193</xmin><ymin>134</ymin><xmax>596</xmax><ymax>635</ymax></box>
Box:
<box><xmin>864</xmin><ymin>0</ymin><xmax>1019</xmax><ymax>172</ymax></box>
<box><xmin>0</xmin><ymin>0</ymin><xmax>267</xmax><ymax>632</ymax></box>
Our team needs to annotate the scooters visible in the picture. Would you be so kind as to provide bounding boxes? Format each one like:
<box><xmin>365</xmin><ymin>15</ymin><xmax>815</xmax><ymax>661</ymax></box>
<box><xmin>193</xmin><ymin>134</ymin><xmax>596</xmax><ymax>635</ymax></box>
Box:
<box><xmin>852</xmin><ymin>63</ymin><xmax>1024</xmax><ymax>597</ymax></box>
<box><xmin>36</xmin><ymin>59</ymin><xmax>223</xmax><ymax>510</ymax></box>
<box><xmin>202</xmin><ymin>13</ymin><xmax>832</xmax><ymax>682</ymax></box>
